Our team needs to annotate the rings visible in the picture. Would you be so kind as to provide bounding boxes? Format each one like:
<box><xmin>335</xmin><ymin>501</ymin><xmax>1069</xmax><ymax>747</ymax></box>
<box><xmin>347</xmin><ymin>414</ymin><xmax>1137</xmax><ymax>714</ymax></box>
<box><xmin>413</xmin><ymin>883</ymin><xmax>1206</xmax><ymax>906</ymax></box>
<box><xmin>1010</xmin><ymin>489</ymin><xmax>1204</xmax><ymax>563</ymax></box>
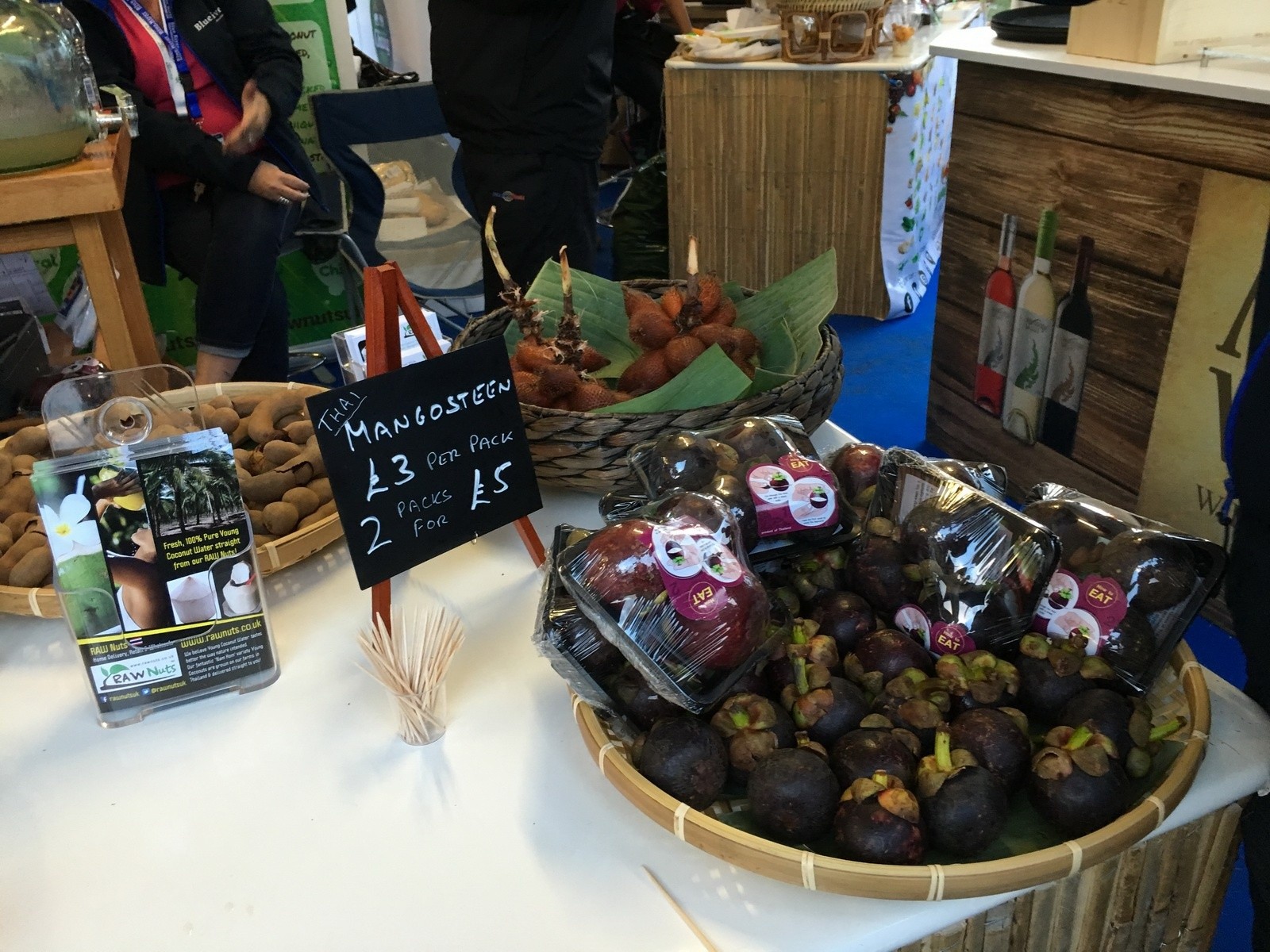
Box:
<box><xmin>278</xmin><ymin>196</ymin><xmax>289</xmax><ymax>205</ymax></box>
<box><xmin>247</xmin><ymin>134</ymin><xmax>252</xmax><ymax>144</ymax></box>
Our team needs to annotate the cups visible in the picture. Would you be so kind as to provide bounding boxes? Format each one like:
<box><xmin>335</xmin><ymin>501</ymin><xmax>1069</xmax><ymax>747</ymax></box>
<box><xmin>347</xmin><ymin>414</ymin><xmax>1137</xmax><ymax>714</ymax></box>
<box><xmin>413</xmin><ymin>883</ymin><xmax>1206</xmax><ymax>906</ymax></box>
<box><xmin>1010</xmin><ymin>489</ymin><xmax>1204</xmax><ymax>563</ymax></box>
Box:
<box><xmin>885</xmin><ymin>0</ymin><xmax>921</xmax><ymax>57</ymax></box>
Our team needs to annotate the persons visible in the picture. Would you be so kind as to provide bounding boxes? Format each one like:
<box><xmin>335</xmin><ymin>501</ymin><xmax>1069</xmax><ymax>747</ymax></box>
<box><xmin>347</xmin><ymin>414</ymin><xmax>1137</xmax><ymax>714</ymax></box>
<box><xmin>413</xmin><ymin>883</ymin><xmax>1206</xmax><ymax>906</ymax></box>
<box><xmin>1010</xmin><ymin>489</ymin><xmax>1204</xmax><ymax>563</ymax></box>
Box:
<box><xmin>33</xmin><ymin>0</ymin><xmax>330</xmax><ymax>384</ymax></box>
<box><xmin>616</xmin><ymin>0</ymin><xmax>693</xmax><ymax>35</ymax></box>
<box><xmin>428</xmin><ymin>0</ymin><xmax>618</xmax><ymax>315</ymax></box>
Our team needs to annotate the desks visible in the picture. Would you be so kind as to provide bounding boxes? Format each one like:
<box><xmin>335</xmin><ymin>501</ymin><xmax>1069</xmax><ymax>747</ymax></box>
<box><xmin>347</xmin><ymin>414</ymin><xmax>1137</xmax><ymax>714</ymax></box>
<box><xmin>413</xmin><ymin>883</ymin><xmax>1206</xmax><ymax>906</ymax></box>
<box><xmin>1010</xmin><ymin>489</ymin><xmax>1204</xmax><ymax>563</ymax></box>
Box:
<box><xmin>0</xmin><ymin>438</ymin><xmax>1270</xmax><ymax>952</ymax></box>
<box><xmin>0</xmin><ymin>114</ymin><xmax>172</xmax><ymax>434</ymax></box>
<box><xmin>664</xmin><ymin>0</ymin><xmax>985</xmax><ymax>322</ymax></box>
<box><xmin>928</xmin><ymin>25</ymin><xmax>1270</xmax><ymax>643</ymax></box>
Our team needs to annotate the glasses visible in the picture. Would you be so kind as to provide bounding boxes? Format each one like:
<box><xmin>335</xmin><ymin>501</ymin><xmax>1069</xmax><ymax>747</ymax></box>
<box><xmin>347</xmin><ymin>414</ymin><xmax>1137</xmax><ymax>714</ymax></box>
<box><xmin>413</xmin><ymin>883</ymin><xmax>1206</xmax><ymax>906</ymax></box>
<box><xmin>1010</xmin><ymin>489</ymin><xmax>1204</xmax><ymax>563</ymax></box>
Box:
<box><xmin>782</xmin><ymin>10</ymin><xmax>872</xmax><ymax>59</ymax></box>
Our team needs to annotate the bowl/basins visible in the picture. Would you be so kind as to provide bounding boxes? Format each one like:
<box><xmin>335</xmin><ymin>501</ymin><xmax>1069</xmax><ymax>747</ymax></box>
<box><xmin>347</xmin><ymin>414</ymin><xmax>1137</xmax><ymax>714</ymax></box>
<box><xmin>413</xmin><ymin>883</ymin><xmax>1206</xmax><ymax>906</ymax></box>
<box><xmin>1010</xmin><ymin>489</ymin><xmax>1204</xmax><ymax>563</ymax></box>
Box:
<box><xmin>935</xmin><ymin>1</ymin><xmax>981</xmax><ymax>22</ymax></box>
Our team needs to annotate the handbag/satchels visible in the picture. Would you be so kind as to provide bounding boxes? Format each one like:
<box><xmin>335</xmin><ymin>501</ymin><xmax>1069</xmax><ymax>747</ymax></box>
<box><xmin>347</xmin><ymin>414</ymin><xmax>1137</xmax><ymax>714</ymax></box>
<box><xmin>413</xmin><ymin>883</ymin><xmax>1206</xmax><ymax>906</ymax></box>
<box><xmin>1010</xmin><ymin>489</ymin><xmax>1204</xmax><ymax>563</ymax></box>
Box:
<box><xmin>294</xmin><ymin>172</ymin><xmax>350</xmax><ymax>264</ymax></box>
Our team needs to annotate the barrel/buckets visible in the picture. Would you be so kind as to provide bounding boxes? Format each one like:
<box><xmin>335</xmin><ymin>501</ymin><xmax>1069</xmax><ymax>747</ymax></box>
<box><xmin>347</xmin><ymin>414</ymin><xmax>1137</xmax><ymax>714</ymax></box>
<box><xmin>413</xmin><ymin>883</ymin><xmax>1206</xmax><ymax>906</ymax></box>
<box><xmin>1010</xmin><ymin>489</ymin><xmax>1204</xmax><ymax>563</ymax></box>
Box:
<box><xmin>0</xmin><ymin>0</ymin><xmax>139</xmax><ymax>176</ymax></box>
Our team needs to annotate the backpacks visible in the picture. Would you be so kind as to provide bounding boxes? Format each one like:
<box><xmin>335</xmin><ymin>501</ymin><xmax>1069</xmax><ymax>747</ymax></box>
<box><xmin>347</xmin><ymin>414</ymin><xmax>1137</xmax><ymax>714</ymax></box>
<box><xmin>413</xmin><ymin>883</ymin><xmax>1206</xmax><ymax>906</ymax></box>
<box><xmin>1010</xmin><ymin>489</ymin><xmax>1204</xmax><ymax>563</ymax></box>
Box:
<box><xmin>574</xmin><ymin>151</ymin><xmax>669</xmax><ymax>281</ymax></box>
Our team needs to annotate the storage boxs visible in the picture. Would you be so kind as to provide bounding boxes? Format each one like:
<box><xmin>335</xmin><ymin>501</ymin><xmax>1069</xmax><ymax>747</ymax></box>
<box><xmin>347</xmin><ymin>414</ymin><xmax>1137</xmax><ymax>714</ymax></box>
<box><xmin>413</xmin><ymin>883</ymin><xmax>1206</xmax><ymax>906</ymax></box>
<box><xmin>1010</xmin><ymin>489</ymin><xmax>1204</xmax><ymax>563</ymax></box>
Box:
<box><xmin>332</xmin><ymin>325</ymin><xmax>454</xmax><ymax>388</ymax></box>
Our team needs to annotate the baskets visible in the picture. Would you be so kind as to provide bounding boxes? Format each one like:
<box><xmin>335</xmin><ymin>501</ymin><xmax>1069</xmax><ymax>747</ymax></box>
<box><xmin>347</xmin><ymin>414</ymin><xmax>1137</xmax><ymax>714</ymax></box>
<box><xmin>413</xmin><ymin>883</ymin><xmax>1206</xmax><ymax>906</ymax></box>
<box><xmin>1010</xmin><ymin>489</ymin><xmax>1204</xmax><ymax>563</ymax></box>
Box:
<box><xmin>0</xmin><ymin>382</ymin><xmax>344</xmax><ymax>620</ymax></box>
<box><xmin>444</xmin><ymin>277</ymin><xmax>844</xmax><ymax>493</ymax></box>
<box><xmin>570</xmin><ymin>637</ymin><xmax>1211</xmax><ymax>902</ymax></box>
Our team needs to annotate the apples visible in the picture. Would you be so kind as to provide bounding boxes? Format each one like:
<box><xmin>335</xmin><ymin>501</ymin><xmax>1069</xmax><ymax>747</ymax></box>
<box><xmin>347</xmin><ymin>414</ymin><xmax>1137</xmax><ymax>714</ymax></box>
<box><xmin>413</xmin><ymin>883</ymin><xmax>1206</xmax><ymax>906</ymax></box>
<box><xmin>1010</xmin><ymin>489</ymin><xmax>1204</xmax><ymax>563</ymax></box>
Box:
<box><xmin>662</xmin><ymin>571</ymin><xmax>768</xmax><ymax>669</ymax></box>
<box><xmin>584</xmin><ymin>520</ymin><xmax>663</xmax><ymax>601</ymax></box>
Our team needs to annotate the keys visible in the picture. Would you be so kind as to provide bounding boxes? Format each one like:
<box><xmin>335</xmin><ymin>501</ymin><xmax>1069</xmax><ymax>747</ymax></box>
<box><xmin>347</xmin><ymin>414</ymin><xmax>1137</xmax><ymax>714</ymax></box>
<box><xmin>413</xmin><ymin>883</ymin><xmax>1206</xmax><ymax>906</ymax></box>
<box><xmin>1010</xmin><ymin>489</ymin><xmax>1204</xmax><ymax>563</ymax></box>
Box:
<box><xmin>194</xmin><ymin>183</ymin><xmax>205</xmax><ymax>202</ymax></box>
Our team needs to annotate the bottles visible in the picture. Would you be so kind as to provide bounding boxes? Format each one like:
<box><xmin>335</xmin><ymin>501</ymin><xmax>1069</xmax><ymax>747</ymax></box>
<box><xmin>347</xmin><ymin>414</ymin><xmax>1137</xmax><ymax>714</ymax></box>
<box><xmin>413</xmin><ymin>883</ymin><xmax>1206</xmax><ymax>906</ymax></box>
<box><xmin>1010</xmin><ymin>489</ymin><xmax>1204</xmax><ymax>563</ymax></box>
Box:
<box><xmin>1037</xmin><ymin>234</ymin><xmax>1093</xmax><ymax>458</ymax></box>
<box><xmin>1002</xmin><ymin>207</ymin><xmax>1058</xmax><ymax>447</ymax></box>
<box><xmin>34</xmin><ymin>0</ymin><xmax>108</xmax><ymax>142</ymax></box>
<box><xmin>974</xmin><ymin>212</ymin><xmax>1017</xmax><ymax>418</ymax></box>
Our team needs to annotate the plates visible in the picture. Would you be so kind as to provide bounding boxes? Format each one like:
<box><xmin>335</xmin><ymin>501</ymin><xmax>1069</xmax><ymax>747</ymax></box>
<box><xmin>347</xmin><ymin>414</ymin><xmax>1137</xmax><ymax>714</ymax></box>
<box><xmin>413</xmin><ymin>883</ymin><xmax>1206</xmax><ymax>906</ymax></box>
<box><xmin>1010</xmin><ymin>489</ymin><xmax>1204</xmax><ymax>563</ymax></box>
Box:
<box><xmin>990</xmin><ymin>4</ymin><xmax>1083</xmax><ymax>45</ymax></box>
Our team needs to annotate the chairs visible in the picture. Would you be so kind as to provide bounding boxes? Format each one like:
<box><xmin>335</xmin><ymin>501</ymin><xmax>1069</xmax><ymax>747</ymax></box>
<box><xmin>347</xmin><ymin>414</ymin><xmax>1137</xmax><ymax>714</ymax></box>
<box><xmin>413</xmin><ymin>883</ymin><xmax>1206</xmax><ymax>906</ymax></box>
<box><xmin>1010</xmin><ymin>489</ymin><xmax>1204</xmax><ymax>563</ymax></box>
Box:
<box><xmin>292</xmin><ymin>82</ymin><xmax>491</xmax><ymax>339</ymax></box>
